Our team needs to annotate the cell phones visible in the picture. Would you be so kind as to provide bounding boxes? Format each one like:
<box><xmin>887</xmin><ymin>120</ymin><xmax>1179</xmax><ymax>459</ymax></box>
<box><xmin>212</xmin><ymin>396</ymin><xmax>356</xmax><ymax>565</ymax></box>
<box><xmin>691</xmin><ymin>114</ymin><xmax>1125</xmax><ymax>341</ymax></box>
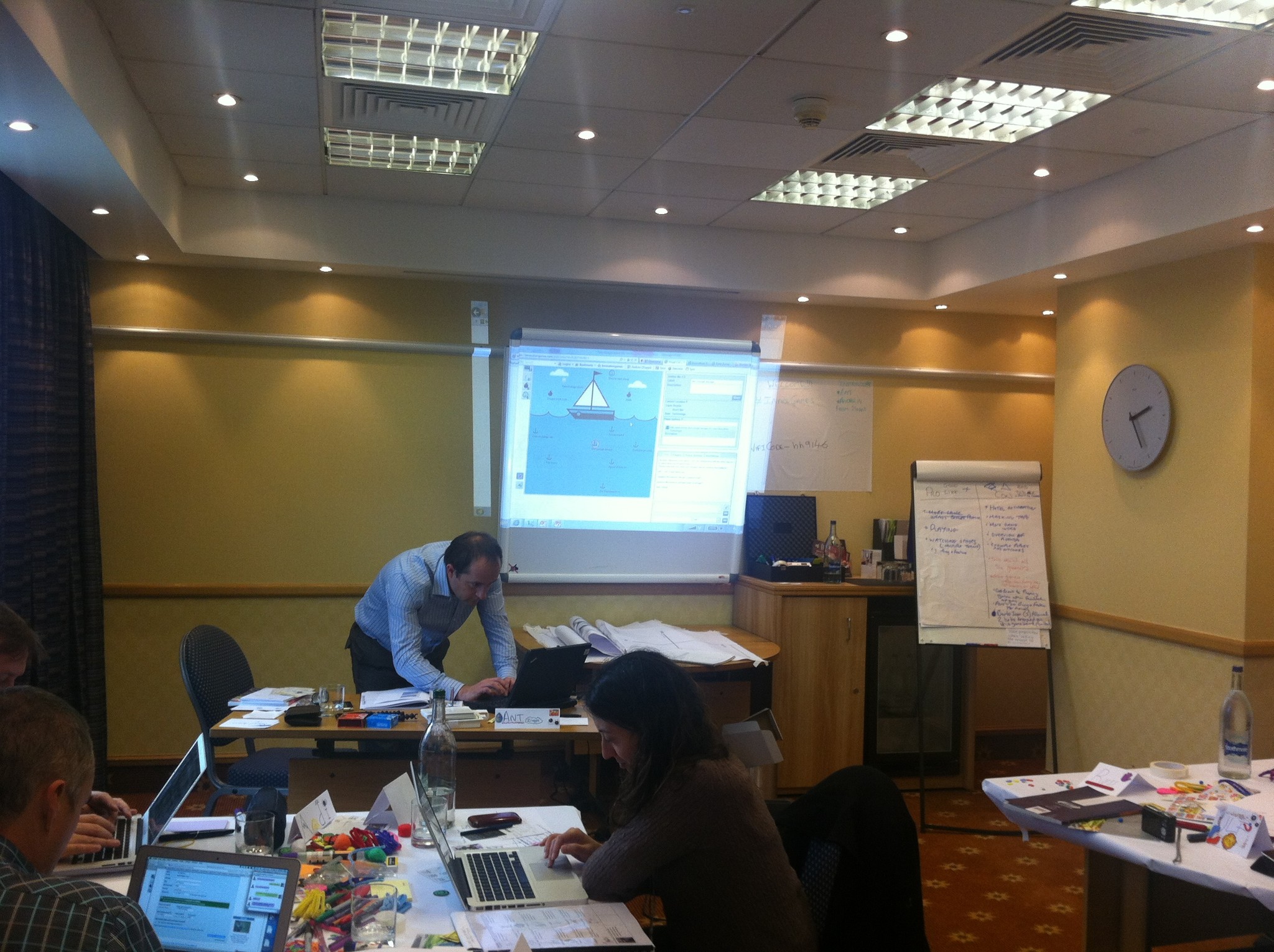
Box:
<box><xmin>342</xmin><ymin>701</ymin><xmax>354</xmax><ymax>711</ymax></box>
<box><xmin>467</xmin><ymin>812</ymin><xmax>522</xmax><ymax>828</ymax></box>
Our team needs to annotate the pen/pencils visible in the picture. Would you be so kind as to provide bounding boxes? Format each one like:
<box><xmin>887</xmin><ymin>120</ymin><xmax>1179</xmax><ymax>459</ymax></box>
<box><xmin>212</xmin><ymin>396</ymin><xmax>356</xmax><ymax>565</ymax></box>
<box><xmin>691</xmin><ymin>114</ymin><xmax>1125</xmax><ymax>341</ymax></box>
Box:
<box><xmin>287</xmin><ymin>885</ymin><xmax>383</xmax><ymax>952</ymax></box>
<box><xmin>461</xmin><ymin>823</ymin><xmax>513</xmax><ymax>836</ymax></box>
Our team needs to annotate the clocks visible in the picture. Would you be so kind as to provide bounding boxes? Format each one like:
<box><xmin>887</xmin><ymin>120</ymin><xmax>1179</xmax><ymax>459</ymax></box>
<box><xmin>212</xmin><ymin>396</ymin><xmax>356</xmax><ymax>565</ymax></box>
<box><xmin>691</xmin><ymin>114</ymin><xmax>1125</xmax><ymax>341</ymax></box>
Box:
<box><xmin>1101</xmin><ymin>363</ymin><xmax>1174</xmax><ymax>473</ymax></box>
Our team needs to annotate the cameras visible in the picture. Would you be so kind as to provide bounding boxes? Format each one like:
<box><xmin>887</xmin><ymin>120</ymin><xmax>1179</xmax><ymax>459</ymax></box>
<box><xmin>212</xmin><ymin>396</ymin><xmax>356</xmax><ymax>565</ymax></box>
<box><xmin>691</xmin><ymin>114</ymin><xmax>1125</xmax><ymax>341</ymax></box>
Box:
<box><xmin>1141</xmin><ymin>804</ymin><xmax>1177</xmax><ymax>843</ymax></box>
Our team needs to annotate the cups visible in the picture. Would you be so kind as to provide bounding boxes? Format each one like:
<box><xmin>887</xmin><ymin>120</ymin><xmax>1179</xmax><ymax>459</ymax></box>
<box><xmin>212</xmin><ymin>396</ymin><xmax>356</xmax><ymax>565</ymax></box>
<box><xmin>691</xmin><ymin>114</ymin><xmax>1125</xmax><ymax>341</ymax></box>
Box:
<box><xmin>235</xmin><ymin>810</ymin><xmax>275</xmax><ymax>857</ymax></box>
<box><xmin>428</xmin><ymin>687</ymin><xmax>454</xmax><ymax>726</ymax></box>
<box><xmin>350</xmin><ymin>883</ymin><xmax>398</xmax><ymax>946</ymax></box>
<box><xmin>410</xmin><ymin>796</ymin><xmax>447</xmax><ymax>848</ymax></box>
<box><xmin>876</xmin><ymin>558</ymin><xmax>912</xmax><ymax>582</ymax></box>
<box><xmin>426</xmin><ymin>787</ymin><xmax>455</xmax><ymax>828</ymax></box>
<box><xmin>319</xmin><ymin>684</ymin><xmax>345</xmax><ymax>711</ymax></box>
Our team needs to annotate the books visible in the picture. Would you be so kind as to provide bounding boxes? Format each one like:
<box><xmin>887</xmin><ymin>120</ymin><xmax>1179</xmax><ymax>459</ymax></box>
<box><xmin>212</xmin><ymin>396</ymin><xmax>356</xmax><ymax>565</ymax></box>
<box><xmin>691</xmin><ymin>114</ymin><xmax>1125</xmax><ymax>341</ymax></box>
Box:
<box><xmin>227</xmin><ymin>681</ymin><xmax>316</xmax><ymax>710</ymax></box>
<box><xmin>359</xmin><ymin>687</ymin><xmax>481</xmax><ymax>728</ymax></box>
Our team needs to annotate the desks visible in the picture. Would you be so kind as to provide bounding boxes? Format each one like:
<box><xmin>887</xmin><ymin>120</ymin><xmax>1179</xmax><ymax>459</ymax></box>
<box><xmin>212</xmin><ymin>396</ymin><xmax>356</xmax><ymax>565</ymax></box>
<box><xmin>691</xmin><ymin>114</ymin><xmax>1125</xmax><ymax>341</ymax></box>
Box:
<box><xmin>205</xmin><ymin>621</ymin><xmax>782</xmax><ymax>790</ymax></box>
<box><xmin>730</xmin><ymin>572</ymin><xmax>1049</xmax><ymax>800</ymax></box>
<box><xmin>982</xmin><ymin>757</ymin><xmax>1273</xmax><ymax>952</ymax></box>
<box><xmin>70</xmin><ymin>803</ymin><xmax>600</xmax><ymax>951</ymax></box>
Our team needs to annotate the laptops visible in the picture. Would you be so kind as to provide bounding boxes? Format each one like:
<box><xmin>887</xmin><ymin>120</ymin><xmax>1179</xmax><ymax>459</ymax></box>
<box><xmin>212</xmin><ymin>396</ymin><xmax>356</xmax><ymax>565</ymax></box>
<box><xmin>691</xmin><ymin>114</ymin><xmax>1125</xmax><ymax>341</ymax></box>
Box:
<box><xmin>462</xmin><ymin>642</ymin><xmax>592</xmax><ymax>715</ymax></box>
<box><xmin>409</xmin><ymin>761</ymin><xmax>590</xmax><ymax>911</ymax></box>
<box><xmin>126</xmin><ymin>843</ymin><xmax>301</xmax><ymax>952</ymax></box>
<box><xmin>52</xmin><ymin>731</ymin><xmax>207</xmax><ymax>878</ymax></box>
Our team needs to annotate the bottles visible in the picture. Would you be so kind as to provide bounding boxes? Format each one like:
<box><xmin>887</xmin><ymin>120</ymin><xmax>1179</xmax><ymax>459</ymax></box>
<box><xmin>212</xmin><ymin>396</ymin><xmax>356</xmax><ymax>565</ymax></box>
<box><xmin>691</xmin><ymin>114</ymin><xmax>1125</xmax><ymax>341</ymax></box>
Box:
<box><xmin>1216</xmin><ymin>664</ymin><xmax>1252</xmax><ymax>779</ymax></box>
<box><xmin>820</xmin><ymin>519</ymin><xmax>842</xmax><ymax>583</ymax></box>
<box><xmin>417</xmin><ymin>689</ymin><xmax>456</xmax><ymax>830</ymax></box>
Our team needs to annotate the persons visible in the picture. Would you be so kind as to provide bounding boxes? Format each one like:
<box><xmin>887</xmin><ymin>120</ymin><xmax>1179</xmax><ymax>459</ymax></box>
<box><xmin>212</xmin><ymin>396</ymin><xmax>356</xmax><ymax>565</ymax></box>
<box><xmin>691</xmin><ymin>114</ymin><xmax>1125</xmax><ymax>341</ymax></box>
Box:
<box><xmin>0</xmin><ymin>608</ymin><xmax>136</xmax><ymax>861</ymax></box>
<box><xmin>534</xmin><ymin>647</ymin><xmax>832</xmax><ymax>951</ymax></box>
<box><xmin>345</xmin><ymin>529</ymin><xmax>519</xmax><ymax>710</ymax></box>
<box><xmin>2</xmin><ymin>684</ymin><xmax>163</xmax><ymax>950</ymax></box>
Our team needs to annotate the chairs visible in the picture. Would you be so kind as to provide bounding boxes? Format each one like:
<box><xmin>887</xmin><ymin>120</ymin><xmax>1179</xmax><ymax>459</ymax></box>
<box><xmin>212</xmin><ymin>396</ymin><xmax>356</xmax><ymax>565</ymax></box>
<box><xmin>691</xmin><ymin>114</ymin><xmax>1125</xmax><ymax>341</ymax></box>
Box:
<box><xmin>178</xmin><ymin>622</ymin><xmax>360</xmax><ymax>817</ymax></box>
<box><xmin>776</xmin><ymin>764</ymin><xmax>933</xmax><ymax>952</ymax></box>
